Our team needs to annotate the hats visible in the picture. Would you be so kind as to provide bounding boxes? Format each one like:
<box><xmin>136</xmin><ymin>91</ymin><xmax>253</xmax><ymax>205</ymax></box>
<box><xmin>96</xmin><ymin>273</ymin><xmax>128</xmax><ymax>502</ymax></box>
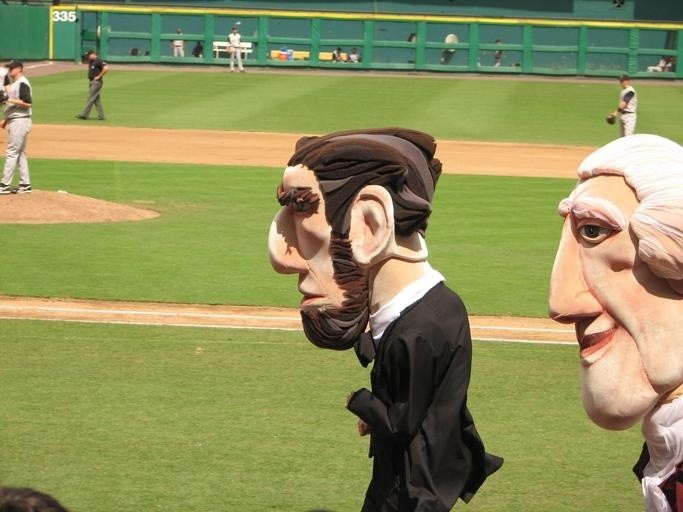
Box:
<box><xmin>618</xmin><ymin>74</ymin><xmax>630</xmax><ymax>81</ymax></box>
<box><xmin>85</xmin><ymin>50</ymin><xmax>94</xmax><ymax>55</ymax></box>
<box><xmin>4</xmin><ymin>60</ymin><xmax>23</xmax><ymax>69</ymax></box>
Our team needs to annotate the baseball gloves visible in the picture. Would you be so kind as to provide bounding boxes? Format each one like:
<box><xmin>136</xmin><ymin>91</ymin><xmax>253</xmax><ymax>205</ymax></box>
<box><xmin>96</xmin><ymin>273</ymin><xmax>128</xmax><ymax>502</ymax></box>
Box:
<box><xmin>606</xmin><ymin>112</ymin><xmax>617</xmax><ymax>124</ymax></box>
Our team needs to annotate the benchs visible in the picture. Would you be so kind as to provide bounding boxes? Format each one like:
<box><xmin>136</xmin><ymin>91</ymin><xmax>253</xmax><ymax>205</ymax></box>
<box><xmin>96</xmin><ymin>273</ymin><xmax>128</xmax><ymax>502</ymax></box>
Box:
<box><xmin>270</xmin><ymin>50</ymin><xmax>348</xmax><ymax>64</ymax></box>
<box><xmin>213</xmin><ymin>40</ymin><xmax>253</xmax><ymax>60</ymax></box>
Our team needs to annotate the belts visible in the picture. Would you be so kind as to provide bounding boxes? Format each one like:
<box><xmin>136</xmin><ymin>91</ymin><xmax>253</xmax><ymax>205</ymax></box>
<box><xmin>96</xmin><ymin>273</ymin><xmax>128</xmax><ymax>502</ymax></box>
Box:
<box><xmin>622</xmin><ymin>111</ymin><xmax>634</xmax><ymax>114</ymax></box>
<box><xmin>8</xmin><ymin>115</ymin><xmax>31</xmax><ymax>120</ymax></box>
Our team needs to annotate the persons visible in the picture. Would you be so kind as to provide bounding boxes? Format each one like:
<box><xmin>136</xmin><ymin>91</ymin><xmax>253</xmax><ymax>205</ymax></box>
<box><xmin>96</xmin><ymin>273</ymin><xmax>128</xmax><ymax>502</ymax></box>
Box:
<box><xmin>191</xmin><ymin>40</ymin><xmax>204</xmax><ymax>58</ymax></box>
<box><xmin>75</xmin><ymin>49</ymin><xmax>109</xmax><ymax>121</ymax></box>
<box><xmin>226</xmin><ymin>25</ymin><xmax>246</xmax><ymax>72</ymax></box>
<box><xmin>607</xmin><ymin>73</ymin><xmax>638</xmax><ymax>136</ymax></box>
<box><xmin>547</xmin><ymin>131</ymin><xmax>683</xmax><ymax>512</ymax></box>
<box><xmin>170</xmin><ymin>27</ymin><xmax>186</xmax><ymax>57</ymax></box>
<box><xmin>0</xmin><ymin>60</ymin><xmax>33</xmax><ymax>195</ymax></box>
<box><xmin>1</xmin><ymin>485</ymin><xmax>71</xmax><ymax>512</ymax></box>
<box><xmin>265</xmin><ymin>124</ymin><xmax>505</xmax><ymax>512</ymax></box>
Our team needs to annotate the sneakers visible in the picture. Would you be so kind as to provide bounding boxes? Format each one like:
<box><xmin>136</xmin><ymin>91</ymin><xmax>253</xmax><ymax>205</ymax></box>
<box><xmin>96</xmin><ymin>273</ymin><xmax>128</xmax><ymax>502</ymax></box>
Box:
<box><xmin>97</xmin><ymin>116</ymin><xmax>106</xmax><ymax>120</ymax></box>
<box><xmin>74</xmin><ymin>114</ymin><xmax>87</xmax><ymax>120</ymax></box>
<box><xmin>11</xmin><ymin>184</ymin><xmax>32</xmax><ymax>193</ymax></box>
<box><xmin>0</xmin><ymin>183</ymin><xmax>12</xmax><ymax>194</ymax></box>
